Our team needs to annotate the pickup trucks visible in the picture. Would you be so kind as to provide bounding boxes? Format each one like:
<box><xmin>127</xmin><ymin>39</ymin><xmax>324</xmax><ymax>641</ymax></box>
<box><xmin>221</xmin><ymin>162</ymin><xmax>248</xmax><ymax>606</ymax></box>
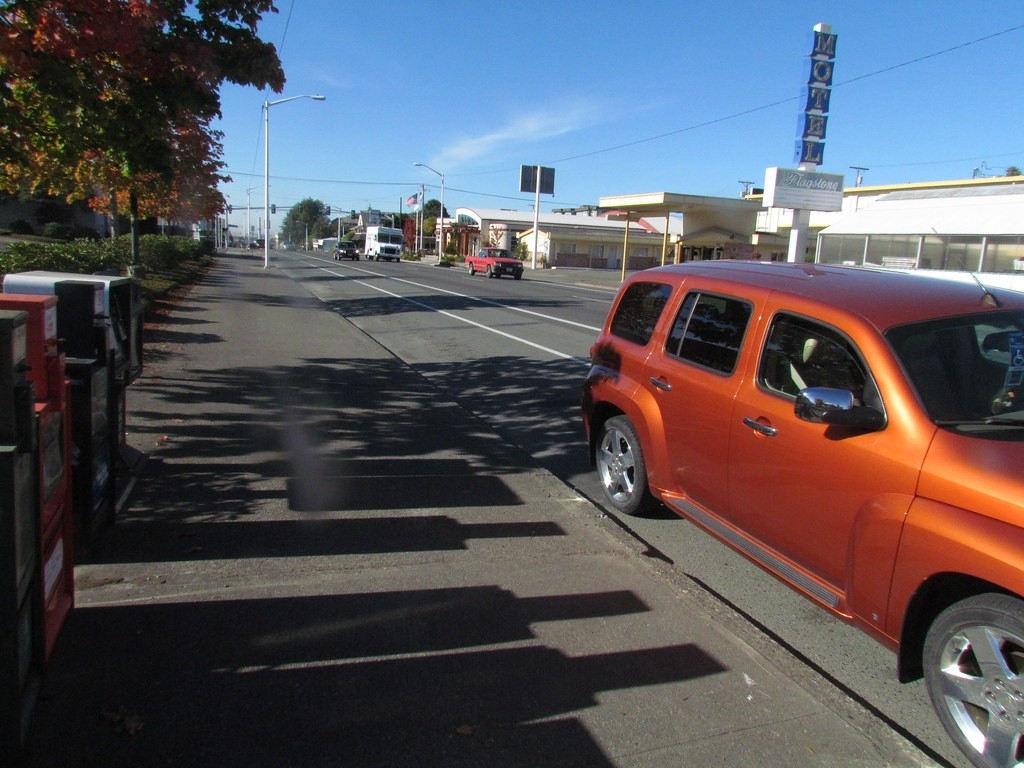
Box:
<box><xmin>465</xmin><ymin>247</ymin><xmax>525</xmax><ymax>279</ymax></box>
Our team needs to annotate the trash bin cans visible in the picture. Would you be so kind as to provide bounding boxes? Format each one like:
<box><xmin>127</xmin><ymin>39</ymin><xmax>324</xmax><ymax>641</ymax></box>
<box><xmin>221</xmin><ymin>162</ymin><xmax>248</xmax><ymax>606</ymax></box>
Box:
<box><xmin>2</xmin><ymin>269</ymin><xmax>143</xmax><ymax>477</ymax></box>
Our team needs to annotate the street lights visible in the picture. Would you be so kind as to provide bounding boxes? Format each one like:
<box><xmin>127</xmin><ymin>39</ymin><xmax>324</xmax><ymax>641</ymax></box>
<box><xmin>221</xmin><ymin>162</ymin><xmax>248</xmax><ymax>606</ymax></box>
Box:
<box><xmin>413</xmin><ymin>163</ymin><xmax>447</xmax><ymax>267</ymax></box>
<box><xmin>296</xmin><ymin>221</ymin><xmax>309</xmax><ymax>251</ymax></box>
<box><xmin>247</xmin><ymin>185</ymin><xmax>272</xmax><ymax>248</ymax></box>
<box><xmin>264</xmin><ymin>94</ymin><xmax>327</xmax><ymax>269</ymax></box>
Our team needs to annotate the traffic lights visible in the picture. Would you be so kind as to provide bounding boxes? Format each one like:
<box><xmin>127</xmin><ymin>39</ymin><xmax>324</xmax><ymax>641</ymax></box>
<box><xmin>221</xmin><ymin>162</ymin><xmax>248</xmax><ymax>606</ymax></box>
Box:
<box><xmin>351</xmin><ymin>209</ymin><xmax>356</xmax><ymax>220</ymax></box>
<box><xmin>325</xmin><ymin>206</ymin><xmax>331</xmax><ymax>216</ymax></box>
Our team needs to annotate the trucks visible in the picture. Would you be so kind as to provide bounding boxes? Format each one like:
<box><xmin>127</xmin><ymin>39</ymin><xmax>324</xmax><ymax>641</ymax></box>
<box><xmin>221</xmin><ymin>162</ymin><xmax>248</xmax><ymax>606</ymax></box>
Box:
<box><xmin>367</xmin><ymin>226</ymin><xmax>404</xmax><ymax>262</ymax></box>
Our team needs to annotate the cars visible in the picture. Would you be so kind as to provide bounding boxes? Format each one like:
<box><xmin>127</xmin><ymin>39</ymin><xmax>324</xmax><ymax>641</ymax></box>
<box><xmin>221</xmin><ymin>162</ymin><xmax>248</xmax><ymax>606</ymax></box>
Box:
<box><xmin>279</xmin><ymin>241</ymin><xmax>296</xmax><ymax>251</ymax></box>
<box><xmin>333</xmin><ymin>241</ymin><xmax>360</xmax><ymax>261</ymax></box>
<box><xmin>581</xmin><ymin>261</ymin><xmax>1022</xmax><ymax>767</ymax></box>
